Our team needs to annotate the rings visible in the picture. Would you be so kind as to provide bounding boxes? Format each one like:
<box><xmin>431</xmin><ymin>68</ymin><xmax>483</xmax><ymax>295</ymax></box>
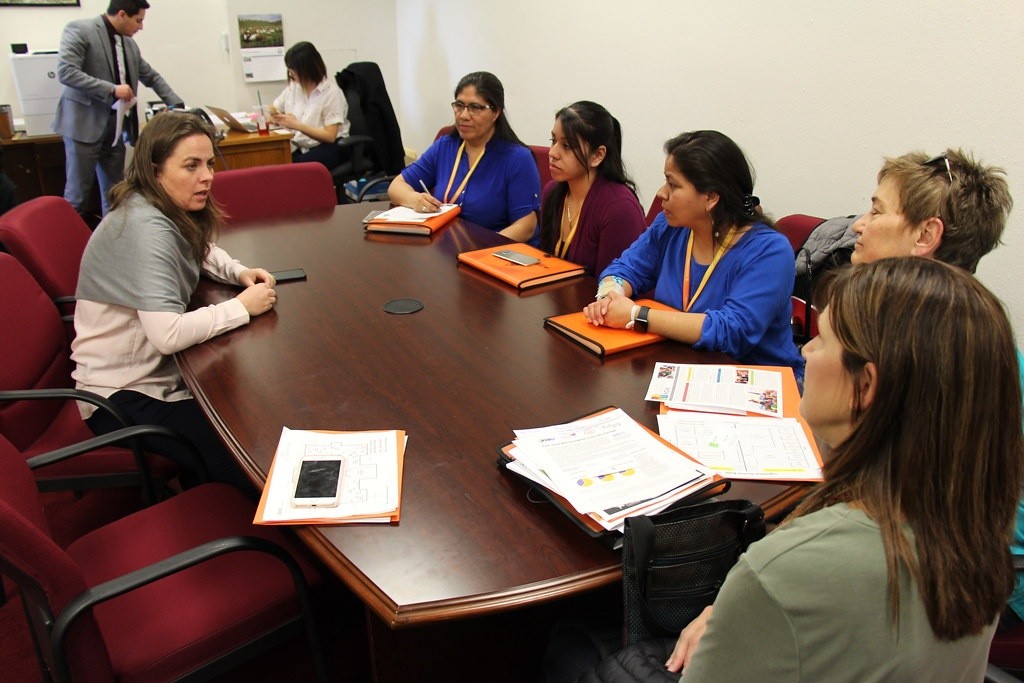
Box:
<box><xmin>423</xmin><ymin>207</ymin><xmax>426</xmax><ymax>210</ymax></box>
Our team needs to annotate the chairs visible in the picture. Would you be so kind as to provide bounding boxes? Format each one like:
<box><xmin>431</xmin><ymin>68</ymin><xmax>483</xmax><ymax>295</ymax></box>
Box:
<box><xmin>207</xmin><ymin>162</ymin><xmax>338</xmax><ymax>223</ymax></box>
<box><xmin>329</xmin><ymin>61</ymin><xmax>407</xmax><ymax>206</ymax></box>
<box><xmin>528</xmin><ymin>144</ymin><xmax>554</xmax><ymax>194</ymax></box>
<box><xmin>0</xmin><ymin>195</ymin><xmax>339</xmax><ymax>683</ymax></box>
<box><xmin>645</xmin><ymin>185</ymin><xmax>778</xmax><ymax>231</ymax></box>
<box><xmin>772</xmin><ymin>214</ymin><xmax>856</xmax><ymax>354</ymax></box>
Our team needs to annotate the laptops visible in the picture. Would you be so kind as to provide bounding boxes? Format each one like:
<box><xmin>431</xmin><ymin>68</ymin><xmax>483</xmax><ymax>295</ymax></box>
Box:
<box><xmin>204</xmin><ymin>105</ymin><xmax>285</xmax><ymax>133</ymax></box>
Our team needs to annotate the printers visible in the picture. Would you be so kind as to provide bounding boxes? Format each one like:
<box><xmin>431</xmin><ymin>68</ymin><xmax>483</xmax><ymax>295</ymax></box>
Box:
<box><xmin>10</xmin><ymin>51</ymin><xmax>68</xmax><ymax>136</ymax></box>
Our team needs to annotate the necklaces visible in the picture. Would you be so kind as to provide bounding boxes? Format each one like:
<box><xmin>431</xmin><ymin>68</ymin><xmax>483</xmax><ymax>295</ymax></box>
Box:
<box><xmin>568</xmin><ymin>194</ymin><xmax>579</xmax><ymax>230</ymax></box>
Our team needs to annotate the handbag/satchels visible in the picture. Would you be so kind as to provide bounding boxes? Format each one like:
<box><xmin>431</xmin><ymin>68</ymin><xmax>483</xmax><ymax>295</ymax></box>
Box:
<box><xmin>623</xmin><ymin>499</ymin><xmax>765</xmax><ymax>648</ymax></box>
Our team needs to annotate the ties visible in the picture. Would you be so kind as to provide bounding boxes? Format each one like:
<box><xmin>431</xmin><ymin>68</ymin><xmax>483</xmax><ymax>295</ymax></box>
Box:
<box><xmin>112</xmin><ymin>33</ymin><xmax>127</xmax><ymax>147</ymax></box>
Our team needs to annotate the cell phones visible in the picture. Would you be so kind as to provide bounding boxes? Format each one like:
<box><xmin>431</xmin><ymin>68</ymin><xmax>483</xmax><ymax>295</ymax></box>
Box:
<box><xmin>290</xmin><ymin>454</ymin><xmax>345</xmax><ymax>509</ymax></box>
<box><xmin>362</xmin><ymin>211</ymin><xmax>384</xmax><ymax>223</ymax></box>
<box><xmin>268</xmin><ymin>269</ymin><xmax>308</xmax><ymax>281</ymax></box>
<box><xmin>492</xmin><ymin>250</ymin><xmax>540</xmax><ymax>266</ymax></box>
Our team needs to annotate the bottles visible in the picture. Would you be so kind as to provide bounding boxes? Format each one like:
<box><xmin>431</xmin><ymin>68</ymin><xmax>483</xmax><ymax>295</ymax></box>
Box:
<box><xmin>0</xmin><ymin>105</ymin><xmax>15</xmax><ymax>139</ymax></box>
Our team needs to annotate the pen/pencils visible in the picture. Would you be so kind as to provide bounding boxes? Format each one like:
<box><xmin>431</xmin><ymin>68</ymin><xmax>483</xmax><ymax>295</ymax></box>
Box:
<box><xmin>257</xmin><ymin>90</ymin><xmax>264</xmax><ymax>115</ymax></box>
<box><xmin>419</xmin><ymin>179</ymin><xmax>431</xmax><ymax>195</ymax></box>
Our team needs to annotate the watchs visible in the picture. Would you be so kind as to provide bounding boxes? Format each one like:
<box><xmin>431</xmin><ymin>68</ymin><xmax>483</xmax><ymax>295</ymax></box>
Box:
<box><xmin>634</xmin><ymin>306</ymin><xmax>651</xmax><ymax>333</ymax></box>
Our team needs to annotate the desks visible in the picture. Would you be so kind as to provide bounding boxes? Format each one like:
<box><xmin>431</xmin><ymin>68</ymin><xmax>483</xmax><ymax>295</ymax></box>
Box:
<box><xmin>0</xmin><ymin>129</ymin><xmax>127</xmax><ymax>233</ymax></box>
<box><xmin>168</xmin><ymin>201</ymin><xmax>839</xmax><ymax>683</ymax></box>
<box><xmin>215</xmin><ymin>112</ymin><xmax>296</xmax><ymax>176</ymax></box>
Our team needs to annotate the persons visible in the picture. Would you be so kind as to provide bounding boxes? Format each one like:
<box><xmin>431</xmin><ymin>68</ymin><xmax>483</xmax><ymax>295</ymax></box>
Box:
<box><xmin>50</xmin><ymin>0</ymin><xmax>185</xmax><ymax>221</ymax></box>
<box><xmin>852</xmin><ymin>148</ymin><xmax>1024</xmax><ymax>634</ymax></box>
<box><xmin>268</xmin><ymin>41</ymin><xmax>351</xmax><ymax>204</ymax></box>
<box><xmin>584</xmin><ymin>129</ymin><xmax>805</xmax><ymax>396</ymax></box>
<box><xmin>543</xmin><ymin>251</ymin><xmax>1024</xmax><ymax>683</ymax></box>
<box><xmin>658</xmin><ymin>366</ymin><xmax>672</xmax><ymax>378</ymax></box>
<box><xmin>71</xmin><ymin>110</ymin><xmax>277</xmax><ymax>548</ymax></box>
<box><xmin>388</xmin><ymin>71</ymin><xmax>542</xmax><ymax>247</ymax></box>
<box><xmin>538</xmin><ymin>101</ymin><xmax>647</xmax><ymax>276</ymax></box>
<box><xmin>749</xmin><ymin>390</ymin><xmax>777</xmax><ymax>412</ymax></box>
<box><xmin>737</xmin><ymin>374</ymin><xmax>748</xmax><ymax>382</ymax></box>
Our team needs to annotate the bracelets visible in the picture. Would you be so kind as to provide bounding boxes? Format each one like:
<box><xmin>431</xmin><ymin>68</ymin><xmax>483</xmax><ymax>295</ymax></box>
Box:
<box><xmin>626</xmin><ymin>306</ymin><xmax>639</xmax><ymax>330</ymax></box>
<box><xmin>112</xmin><ymin>86</ymin><xmax>115</xmax><ymax>98</ymax></box>
<box><xmin>595</xmin><ymin>276</ymin><xmax>624</xmax><ymax>302</ymax></box>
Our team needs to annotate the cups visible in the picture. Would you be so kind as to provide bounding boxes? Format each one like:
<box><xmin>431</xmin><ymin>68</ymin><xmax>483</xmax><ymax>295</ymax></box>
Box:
<box><xmin>253</xmin><ymin>105</ymin><xmax>271</xmax><ymax>136</ymax></box>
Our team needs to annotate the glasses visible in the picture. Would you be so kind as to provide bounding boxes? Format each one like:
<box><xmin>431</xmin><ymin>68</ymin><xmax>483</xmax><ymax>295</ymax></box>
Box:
<box><xmin>923</xmin><ymin>153</ymin><xmax>954</xmax><ymax>184</ymax></box>
<box><xmin>451</xmin><ymin>101</ymin><xmax>496</xmax><ymax>116</ymax></box>
